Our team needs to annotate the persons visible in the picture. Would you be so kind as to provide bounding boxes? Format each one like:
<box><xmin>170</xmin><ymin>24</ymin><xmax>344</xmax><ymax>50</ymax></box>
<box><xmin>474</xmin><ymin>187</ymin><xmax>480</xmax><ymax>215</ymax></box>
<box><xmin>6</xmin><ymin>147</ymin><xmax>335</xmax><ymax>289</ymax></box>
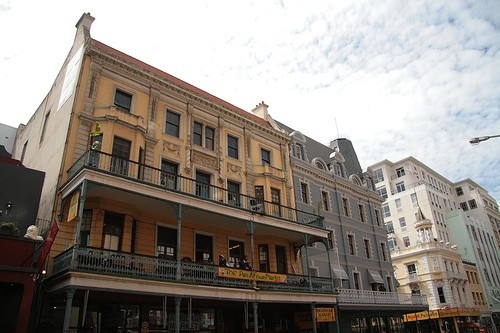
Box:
<box><xmin>219</xmin><ymin>254</ymin><xmax>227</xmax><ymax>267</ymax></box>
<box><xmin>238</xmin><ymin>255</ymin><xmax>255</xmax><ymax>271</ymax></box>
